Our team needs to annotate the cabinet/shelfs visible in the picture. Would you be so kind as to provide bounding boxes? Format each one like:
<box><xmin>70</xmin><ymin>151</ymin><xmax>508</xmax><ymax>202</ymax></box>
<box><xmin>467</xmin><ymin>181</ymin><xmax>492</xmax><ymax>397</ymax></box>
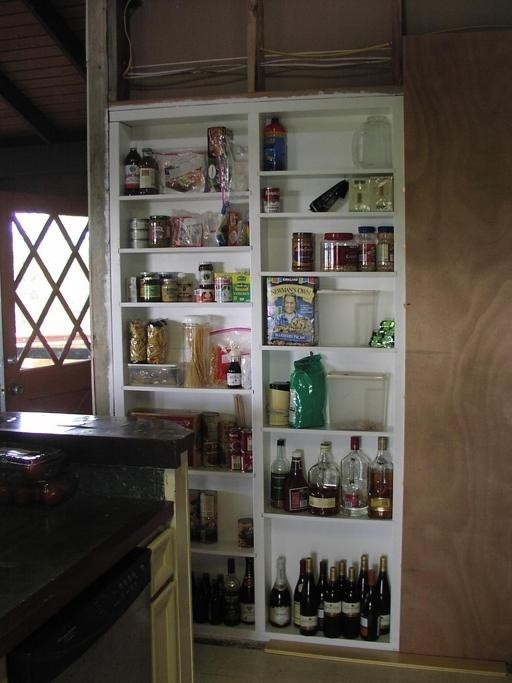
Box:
<box><xmin>108</xmin><ymin>96</ymin><xmax>405</xmax><ymax>650</ymax></box>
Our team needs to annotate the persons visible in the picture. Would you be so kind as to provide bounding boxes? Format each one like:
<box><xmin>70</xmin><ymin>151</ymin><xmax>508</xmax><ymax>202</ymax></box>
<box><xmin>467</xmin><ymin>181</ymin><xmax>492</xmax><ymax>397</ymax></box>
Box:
<box><xmin>273</xmin><ymin>293</ymin><xmax>304</xmax><ymax>331</ymax></box>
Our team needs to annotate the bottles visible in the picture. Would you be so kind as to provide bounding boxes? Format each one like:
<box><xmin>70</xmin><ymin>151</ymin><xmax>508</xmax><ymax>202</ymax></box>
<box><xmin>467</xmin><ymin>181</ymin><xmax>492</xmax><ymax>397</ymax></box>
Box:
<box><xmin>370</xmin><ymin>436</ymin><xmax>393</xmax><ymax>519</ymax></box>
<box><xmin>339</xmin><ymin>436</ymin><xmax>371</xmax><ymax>516</ymax></box>
<box><xmin>270</xmin><ymin>438</ymin><xmax>290</xmax><ymax>511</ymax></box>
<box><xmin>324</xmin><ymin>567</ymin><xmax>342</xmax><ymax>636</ymax></box>
<box><xmin>226</xmin><ymin>356</ymin><xmax>241</xmax><ymax>389</ymax></box>
<box><xmin>308</xmin><ymin>444</ymin><xmax>340</xmax><ymax>516</ymax></box>
<box><xmin>339</xmin><ymin>560</ymin><xmax>347</xmax><ymax>589</ymax></box>
<box><xmin>316</xmin><ymin>559</ymin><xmax>330</xmax><ymax>630</ymax></box>
<box><xmin>269</xmin><ymin>556</ymin><xmax>291</xmax><ymax>627</ymax></box>
<box><xmin>358</xmin><ymin>555</ymin><xmax>369</xmax><ymax>600</ymax></box>
<box><xmin>300</xmin><ymin>558</ymin><xmax>318</xmax><ymax>635</ymax></box>
<box><xmin>343</xmin><ymin>567</ymin><xmax>360</xmax><ymax>638</ymax></box>
<box><xmin>376</xmin><ymin>556</ymin><xmax>391</xmax><ymax>633</ymax></box>
<box><xmin>361</xmin><ymin>570</ymin><xmax>378</xmax><ymax>641</ymax></box>
<box><xmin>285</xmin><ymin>449</ymin><xmax>308</xmax><ymax>512</ymax></box>
<box><xmin>191</xmin><ymin>556</ymin><xmax>254</xmax><ymax>627</ymax></box>
<box><xmin>293</xmin><ymin>559</ymin><xmax>308</xmax><ymax>628</ymax></box>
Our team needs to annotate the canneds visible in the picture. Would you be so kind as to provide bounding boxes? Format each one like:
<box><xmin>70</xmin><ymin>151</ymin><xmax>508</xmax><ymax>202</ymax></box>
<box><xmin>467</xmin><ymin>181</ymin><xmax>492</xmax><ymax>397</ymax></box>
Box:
<box><xmin>160</xmin><ymin>273</ymin><xmax>171</xmax><ymax>279</ymax></box>
<box><xmin>358</xmin><ymin>226</ymin><xmax>376</xmax><ymax>271</ymax></box>
<box><xmin>377</xmin><ymin>225</ymin><xmax>393</xmax><ymax>272</ymax></box>
<box><xmin>160</xmin><ymin>279</ymin><xmax>178</xmax><ymax>301</ymax></box>
<box><xmin>199</xmin><ymin>263</ymin><xmax>214</xmax><ymax>288</ymax></box>
<box><xmin>176</xmin><ymin>273</ymin><xmax>194</xmax><ymax>301</ymax></box>
<box><xmin>214</xmin><ymin>277</ymin><xmax>233</xmax><ymax>302</ymax></box>
<box><xmin>124</xmin><ymin>142</ymin><xmax>139</xmax><ymax>196</ymax></box>
<box><xmin>238</xmin><ymin>518</ymin><xmax>254</xmax><ymax>548</ymax></box>
<box><xmin>265</xmin><ymin>116</ymin><xmax>286</xmax><ymax>170</ymax></box>
<box><xmin>139</xmin><ymin>272</ymin><xmax>160</xmax><ymax>302</ymax></box>
<box><xmin>149</xmin><ymin>215</ymin><xmax>172</xmax><ymax>247</ymax></box>
<box><xmin>201</xmin><ymin>411</ymin><xmax>253</xmax><ymax>473</ymax></box>
<box><xmin>320</xmin><ymin>233</ymin><xmax>356</xmax><ymax>271</ymax></box>
<box><xmin>263</xmin><ymin>185</ymin><xmax>280</xmax><ymax>212</ymax></box>
<box><xmin>269</xmin><ymin>382</ymin><xmax>289</xmax><ymax>426</ymax></box>
<box><xmin>197</xmin><ymin>289</ymin><xmax>214</xmax><ymax>302</ymax></box>
<box><xmin>139</xmin><ymin>149</ymin><xmax>157</xmax><ymax>195</ymax></box>
<box><xmin>190</xmin><ymin>488</ymin><xmax>218</xmax><ymax>544</ymax></box>
<box><xmin>129</xmin><ymin>218</ymin><xmax>150</xmax><ymax>248</ymax></box>
<box><xmin>291</xmin><ymin>232</ymin><xmax>315</xmax><ymax>270</ymax></box>
